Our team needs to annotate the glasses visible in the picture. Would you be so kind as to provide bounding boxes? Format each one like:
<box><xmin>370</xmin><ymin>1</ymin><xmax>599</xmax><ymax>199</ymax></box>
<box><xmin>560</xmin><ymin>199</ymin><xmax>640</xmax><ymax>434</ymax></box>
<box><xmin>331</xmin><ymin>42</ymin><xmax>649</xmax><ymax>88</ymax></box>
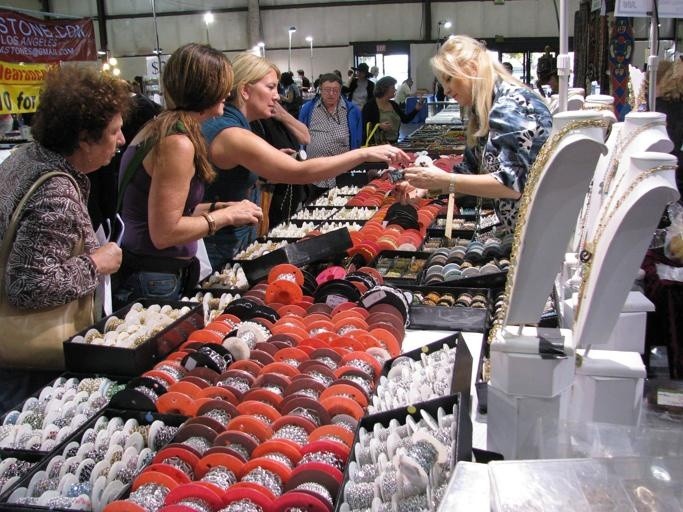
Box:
<box><xmin>319</xmin><ymin>87</ymin><xmax>342</xmax><ymax>95</ymax></box>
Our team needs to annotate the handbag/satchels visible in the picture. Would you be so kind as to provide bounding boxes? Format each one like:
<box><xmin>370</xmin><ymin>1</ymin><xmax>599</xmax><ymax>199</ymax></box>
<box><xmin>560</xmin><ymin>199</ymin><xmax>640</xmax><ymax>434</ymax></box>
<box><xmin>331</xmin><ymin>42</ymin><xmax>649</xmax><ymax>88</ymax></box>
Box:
<box><xmin>0</xmin><ymin>222</ymin><xmax>94</xmax><ymax>372</ymax></box>
<box><xmin>287</xmin><ymin>95</ymin><xmax>303</xmax><ymax>112</ymax></box>
<box><xmin>114</xmin><ymin>268</ymin><xmax>183</xmax><ymax>310</ymax></box>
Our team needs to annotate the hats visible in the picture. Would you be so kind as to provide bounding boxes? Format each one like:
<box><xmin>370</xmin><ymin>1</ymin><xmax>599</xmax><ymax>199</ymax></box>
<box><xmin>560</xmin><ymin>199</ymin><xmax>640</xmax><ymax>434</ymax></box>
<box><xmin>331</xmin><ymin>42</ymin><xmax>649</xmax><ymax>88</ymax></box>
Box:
<box><xmin>350</xmin><ymin>63</ymin><xmax>373</xmax><ymax>78</ymax></box>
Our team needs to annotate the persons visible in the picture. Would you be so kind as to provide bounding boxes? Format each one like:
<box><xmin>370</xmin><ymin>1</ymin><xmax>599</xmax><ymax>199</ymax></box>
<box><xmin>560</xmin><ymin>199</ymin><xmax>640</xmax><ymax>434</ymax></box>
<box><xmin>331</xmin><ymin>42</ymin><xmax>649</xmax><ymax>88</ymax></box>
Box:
<box><xmin>634</xmin><ymin>38</ymin><xmax>681</xmax><ymax>385</ymax></box>
<box><xmin>1</xmin><ymin>64</ymin><xmax>134</xmax><ymax>362</ymax></box>
<box><xmin>86</xmin><ymin>41</ymin><xmax>411</xmax><ymax>318</ymax></box>
<box><xmin>400</xmin><ymin>35</ymin><xmax>552</xmax><ymax>241</ymax></box>
